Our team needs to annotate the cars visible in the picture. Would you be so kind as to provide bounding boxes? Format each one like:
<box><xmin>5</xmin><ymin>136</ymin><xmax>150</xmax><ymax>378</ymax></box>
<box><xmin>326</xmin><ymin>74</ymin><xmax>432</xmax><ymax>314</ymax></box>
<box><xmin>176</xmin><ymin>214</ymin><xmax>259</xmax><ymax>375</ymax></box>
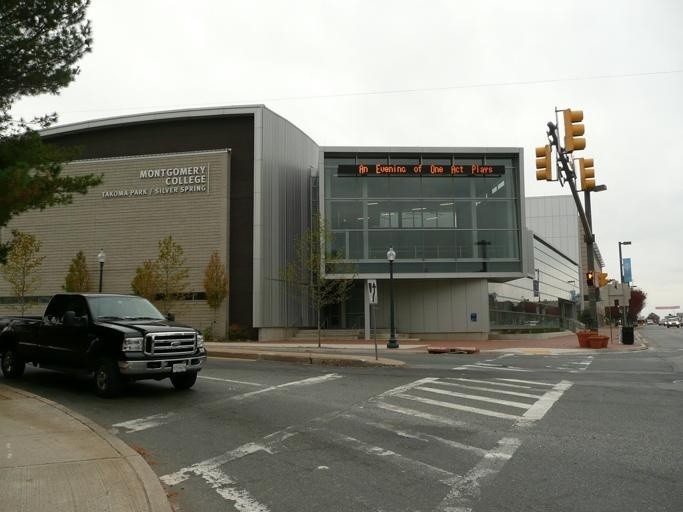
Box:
<box><xmin>644</xmin><ymin>316</ymin><xmax>682</xmax><ymax>329</ymax></box>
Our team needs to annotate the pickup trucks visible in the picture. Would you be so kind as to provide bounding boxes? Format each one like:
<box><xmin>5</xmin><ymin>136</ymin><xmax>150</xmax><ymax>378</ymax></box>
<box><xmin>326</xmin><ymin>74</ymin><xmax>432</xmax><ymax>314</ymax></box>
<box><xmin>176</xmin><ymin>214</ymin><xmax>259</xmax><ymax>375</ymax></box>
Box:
<box><xmin>0</xmin><ymin>291</ymin><xmax>207</xmax><ymax>399</ymax></box>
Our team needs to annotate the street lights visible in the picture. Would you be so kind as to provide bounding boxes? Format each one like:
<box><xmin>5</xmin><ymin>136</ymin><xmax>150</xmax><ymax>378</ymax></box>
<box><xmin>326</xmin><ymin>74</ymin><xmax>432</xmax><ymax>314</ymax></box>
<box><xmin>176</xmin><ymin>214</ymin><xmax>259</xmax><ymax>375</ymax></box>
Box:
<box><xmin>583</xmin><ymin>182</ymin><xmax>609</xmax><ymax>333</ymax></box>
<box><xmin>618</xmin><ymin>241</ymin><xmax>631</xmax><ymax>325</ymax></box>
<box><xmin>386</xmin><ymin>245</ymin><xmax>399</xmax><ymax>349</ymax></box>
<box><xmin>96</xmin><ymin>249</ymin><xmax>107</xmax><ymax>293</ymax></box>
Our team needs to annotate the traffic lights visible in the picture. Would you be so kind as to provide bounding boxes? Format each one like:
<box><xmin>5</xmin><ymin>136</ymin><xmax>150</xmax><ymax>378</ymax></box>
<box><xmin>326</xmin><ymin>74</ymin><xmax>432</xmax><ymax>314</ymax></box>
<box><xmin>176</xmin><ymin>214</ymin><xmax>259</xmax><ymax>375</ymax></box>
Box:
<box><xmin>562</xmin><ymin>108</ymin><xmax>585</xmax><ymax>153</ymax></box>
<box><xmin>578</xmin><ymin>157</ymin><xmax>595</xmax><ymax>190</ymax></box>
<box><xmin>585</xmin><ymin>271</ymin><xmax>594</xmax><ymax>287</ymax></box>
<box><xmin>534</xmin><ymin>145</ymin><xmax>551</xmax><ymax>181</ymax></box>
<box><xmin>598</xmin><ymin>272</ymin><xmax>607</xmax><ymax>287</ymax></box>
